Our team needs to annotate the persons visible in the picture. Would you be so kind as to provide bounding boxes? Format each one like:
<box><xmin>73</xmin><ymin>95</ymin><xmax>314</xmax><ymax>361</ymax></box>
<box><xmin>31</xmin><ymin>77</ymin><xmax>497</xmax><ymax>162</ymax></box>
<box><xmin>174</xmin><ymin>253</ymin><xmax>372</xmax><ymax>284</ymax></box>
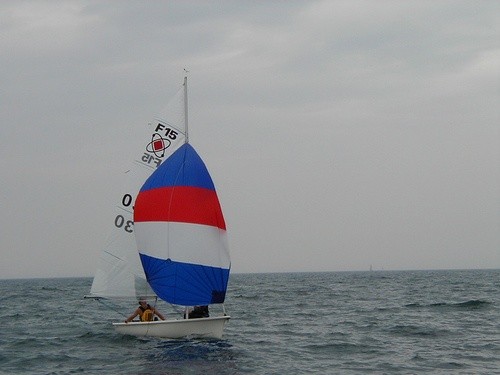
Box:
<box><xmin>123</xmin><ymin>296</ymin><xmax>165</xmax><ymax>323</ymax></box>
<box><xmin>184</xmin><ymin>305</ymin><xmax>209</xmax><ymax>319</ymax></box>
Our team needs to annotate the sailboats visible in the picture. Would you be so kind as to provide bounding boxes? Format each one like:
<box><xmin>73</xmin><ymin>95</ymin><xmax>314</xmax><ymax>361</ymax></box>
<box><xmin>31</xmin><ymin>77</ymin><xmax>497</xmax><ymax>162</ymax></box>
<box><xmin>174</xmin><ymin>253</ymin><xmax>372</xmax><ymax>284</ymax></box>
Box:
<box><xmin>83</xmin><ymin>67</ymin><xmax>231</xmax><ymax>341</ymax></box>
